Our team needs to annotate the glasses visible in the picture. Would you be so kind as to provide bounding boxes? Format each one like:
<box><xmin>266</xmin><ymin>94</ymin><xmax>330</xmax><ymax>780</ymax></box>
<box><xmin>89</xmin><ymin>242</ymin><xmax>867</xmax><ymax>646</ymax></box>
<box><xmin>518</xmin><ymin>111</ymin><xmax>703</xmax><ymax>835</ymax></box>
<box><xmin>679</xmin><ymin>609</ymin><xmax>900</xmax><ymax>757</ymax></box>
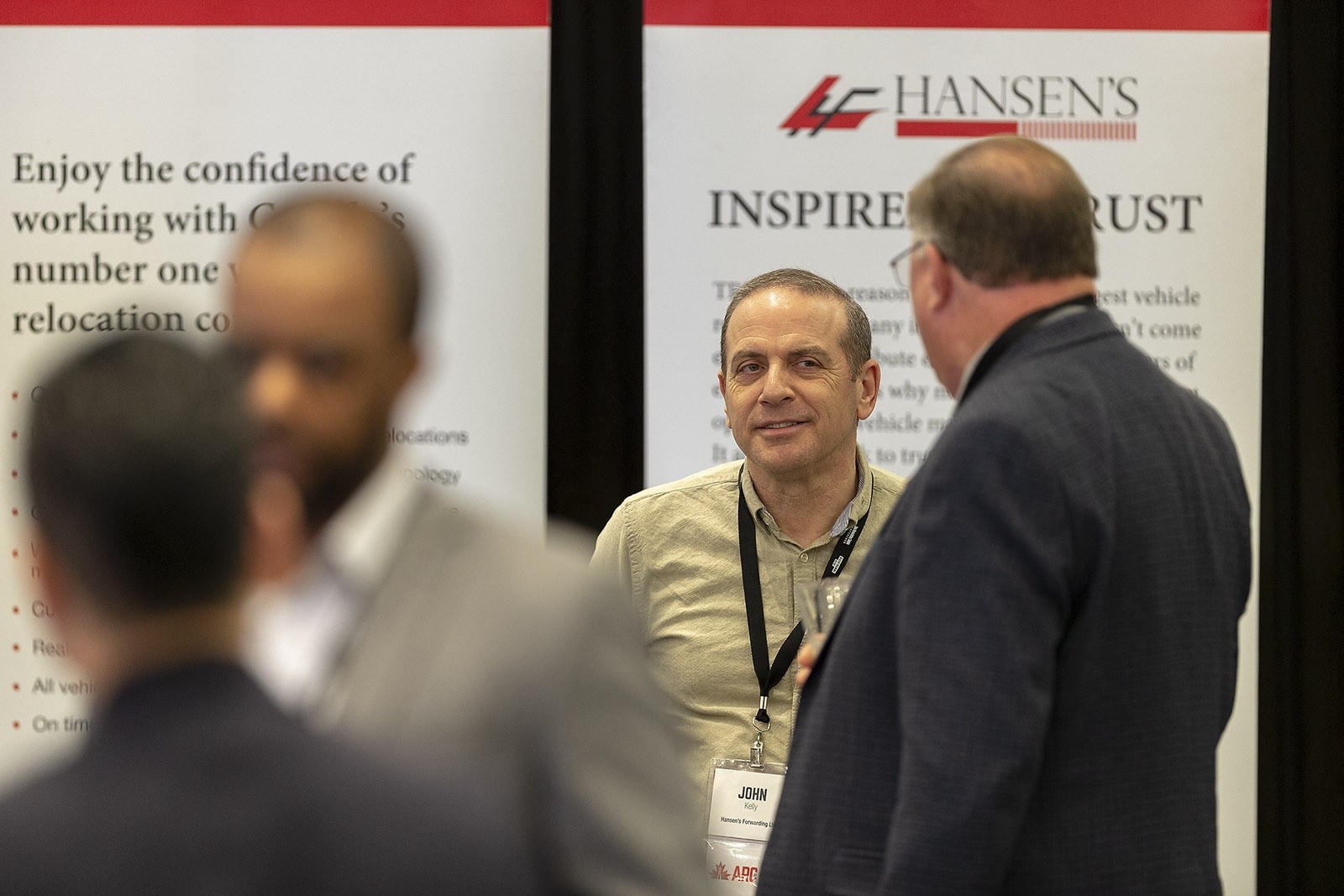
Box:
<box><xmin>890</xmin><ymin>240</ymin><xmax>947</xmax><ymax>289</ymax></box>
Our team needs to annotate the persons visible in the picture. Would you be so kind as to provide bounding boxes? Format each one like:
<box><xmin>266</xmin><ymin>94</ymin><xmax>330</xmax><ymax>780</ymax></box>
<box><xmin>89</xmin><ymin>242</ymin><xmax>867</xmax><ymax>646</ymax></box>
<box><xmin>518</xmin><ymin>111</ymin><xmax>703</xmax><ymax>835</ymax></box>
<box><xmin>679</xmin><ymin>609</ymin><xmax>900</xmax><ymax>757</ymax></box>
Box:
<box><xmin>218</xmin><ymin>189</ymin><xmax>716</xmax><ymax>896</ymax></box>
<box><xmin>591</xmin><ymin>267</ymin><xmax>913</xmax><ymax>896</ymax></box>
<box><xmin>748</xmin><ymin>136</ymin><xmax>1254</xmax><ymax>896</ymax></box>
<box><xmin>0</xmin><ymin>329</ymin><xmax>548</xmax><ymax>896</ymax></box>
